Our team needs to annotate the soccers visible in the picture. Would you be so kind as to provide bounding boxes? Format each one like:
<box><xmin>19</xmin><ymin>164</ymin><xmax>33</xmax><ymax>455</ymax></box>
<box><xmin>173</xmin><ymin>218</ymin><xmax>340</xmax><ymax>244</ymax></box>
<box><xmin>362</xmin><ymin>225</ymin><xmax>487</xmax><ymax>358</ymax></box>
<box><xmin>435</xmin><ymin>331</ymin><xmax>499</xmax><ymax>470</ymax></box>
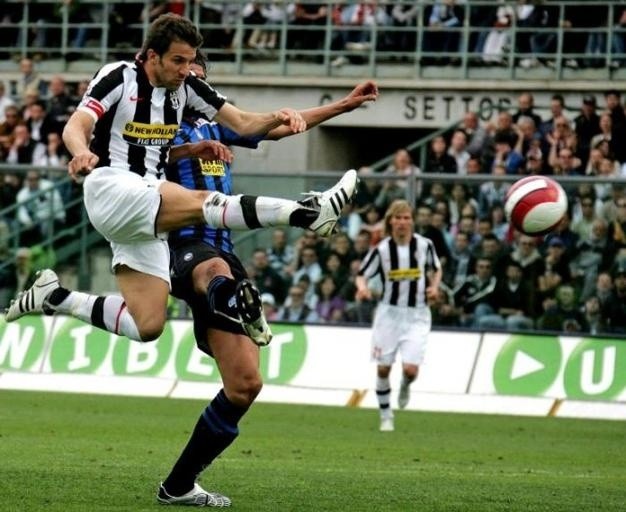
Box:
<box><xmin>502</xmin><ymin>175</ymin><xmax>570</xmax><ymax>237</ymax></box>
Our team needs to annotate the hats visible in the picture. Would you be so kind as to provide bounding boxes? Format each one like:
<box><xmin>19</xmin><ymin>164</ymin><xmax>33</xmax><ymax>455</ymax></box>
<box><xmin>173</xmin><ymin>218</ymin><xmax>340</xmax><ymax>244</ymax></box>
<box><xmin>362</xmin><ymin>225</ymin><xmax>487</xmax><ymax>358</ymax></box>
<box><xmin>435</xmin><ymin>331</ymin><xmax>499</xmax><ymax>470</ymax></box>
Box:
<box><xmin>582</xmin><ymin>94</ymin><xmax>596</xmax><ymax>106</ymax></box>
<box><xmin>546</xmin><ymin>236</ymin><xmax>566</xmax><ymax>246</ymax></box>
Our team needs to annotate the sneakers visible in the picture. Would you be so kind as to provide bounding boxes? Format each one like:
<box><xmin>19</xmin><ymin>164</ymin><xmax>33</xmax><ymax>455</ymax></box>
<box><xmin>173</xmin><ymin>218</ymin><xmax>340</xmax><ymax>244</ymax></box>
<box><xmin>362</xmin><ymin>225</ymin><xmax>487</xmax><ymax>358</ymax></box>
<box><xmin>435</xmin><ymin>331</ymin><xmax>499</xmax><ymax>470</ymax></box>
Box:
<box><xmin>3</xmin><ymin>268</ymin><xmax>62</xmax><ymax>324</ymax></box>
<box><xmin>306</xmin><ymin>167</ymin><xmax>361</xmax><ymax>240</ymax></box>
<box><xmin>379</xmin><ymin>406</ymin><xmax>395</xmax><ymax>432</ymax></box>
<box><xmin>397</xmin><ymin>377</ymin><xmax>410</xmax><ymax>410</ymax></box>
<box><xmin>156</xmin><ymin>479</ymin><xmax>232</xmax><ymax>508</ymax></box>
<box><xmin>234</xmin><ymin>279</ymin><xmax>272</xmax><ymax>348</ymax></box>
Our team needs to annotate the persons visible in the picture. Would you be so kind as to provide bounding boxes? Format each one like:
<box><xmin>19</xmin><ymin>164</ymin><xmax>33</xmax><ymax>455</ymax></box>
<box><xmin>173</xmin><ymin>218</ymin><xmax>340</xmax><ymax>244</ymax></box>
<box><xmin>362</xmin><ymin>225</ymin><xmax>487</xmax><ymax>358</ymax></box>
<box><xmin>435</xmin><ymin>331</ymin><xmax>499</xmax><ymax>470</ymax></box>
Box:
<box><xmin>356</xmin><ymin>200</ymin><xmax>444</xmax><ymax>433</ymax></box>
<box><xmin>244</xmin><ymin>94</ymin><xmax>626</xmax><ymax>339</ymax></box>
<box><xmin>0</xmin><ymin>57</ymin><xmax>89</xmax><ymax>307</ymax></box>
<box><xmin>5</xmin><ymin>13</ymin><xmax>363</xmax><ymax>342</ymax></box>
<box><xmin>156</xmin><ymin>48</ymin><xmax>378</xmax><ymax>508</ymax></box>
